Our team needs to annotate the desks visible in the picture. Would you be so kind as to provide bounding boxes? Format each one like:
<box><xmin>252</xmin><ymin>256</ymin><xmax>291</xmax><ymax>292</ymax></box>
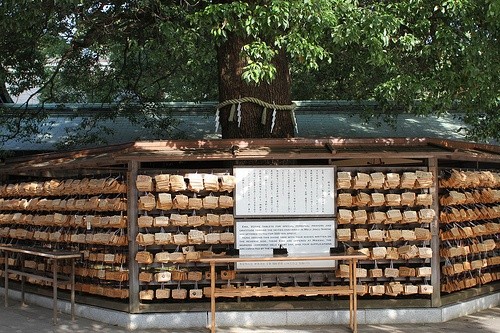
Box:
<box><xmin>0</xmin><ymin>245</ymin><xmax>80</xmax><ymax>325</ymax></box>
<box><xmin>200</xmin><ymin>250</ymin><xmax>367</xmax><ymax>333</ymax></box>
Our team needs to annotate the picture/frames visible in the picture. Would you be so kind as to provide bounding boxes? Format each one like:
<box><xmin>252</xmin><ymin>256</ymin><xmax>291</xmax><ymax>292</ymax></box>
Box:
<box><xmin>233</xmin><ymin>220</ymin><xmax>338</xmax><ymax>272</ymax></box>
<box><xmin>233</xmin><ymin>166</ymin><xmax>336</xmax><ymax>218</ymax></box>
